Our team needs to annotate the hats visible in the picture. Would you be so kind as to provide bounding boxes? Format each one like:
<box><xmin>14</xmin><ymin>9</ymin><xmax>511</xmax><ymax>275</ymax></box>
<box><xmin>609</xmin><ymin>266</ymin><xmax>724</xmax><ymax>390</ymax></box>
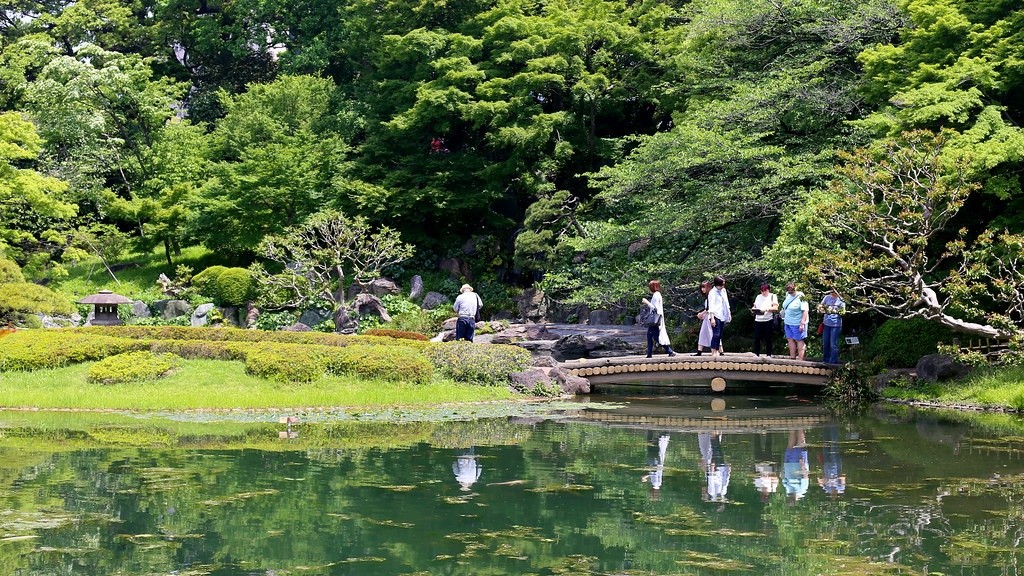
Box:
<box><xmin>460</xmin><ymin>488</ymin><xmax>471</xmax><ymax>492</ymax></box>
<box><xmin>460</xmin><ymin>284</ymin><xmax>473</xmax><ymax>293</ymax></box>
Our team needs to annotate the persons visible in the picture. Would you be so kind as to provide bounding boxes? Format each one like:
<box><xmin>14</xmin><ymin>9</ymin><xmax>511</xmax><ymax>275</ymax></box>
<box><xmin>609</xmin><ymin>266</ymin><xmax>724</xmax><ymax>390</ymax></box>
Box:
<box><xmin>782</xmin><ymin>282</ymin><xmax>809</xmax><ymax>360</ymax></box>
<box><xmin>752</xmin><ymin>433</ymin><xmax>779</xmax><ymax>499</ymax></box>
<box><xmin>749</xmin><ymin>284</ymin><xmax>779</xmax><ymax>356</ymax></box>
<box><xmin>690</xmin><ymin>280</ymin><xmax>724</xmax><ymax>357</ymax></box>
<box><xmin>708</xmin><ymin>276</ymin><xmax>731</xmax><ymax>356</ymax></box>
<box><xmin>818</xmin><ymin>424</ymin><xmax>846</xmax><ymax>500</ymax></box>
<box><xmin>642</xmin><ymin>281</ymin><xmax>676</xmax><ymax>358</ymax></box>
<box><xmin>780</xmin><ymin>428</ymin><xmax>808</xmax><ymax>507</ymax></box>
<box><xmin>453</xmin><ymin>284</ymin><xmax>483</xmax><ymax>343</ymax></box>
<box><xmin>453</xmin><ymin>447</ymin><xmax>481</xmax><ymax>492</ymax></box>
<box><xmin>821</xmin><ymin>290</ymin><xmax>845</xmax><ymax>364</ymax></box>
<box><xmin>698</xmin><ymin>433</ymin><xmax>731</xmax><ymax>512</ymax></box>
<box><xmin>641</xmin><ymin>430</ymin><xmax>670</xmax><ymax>499</ymax></box>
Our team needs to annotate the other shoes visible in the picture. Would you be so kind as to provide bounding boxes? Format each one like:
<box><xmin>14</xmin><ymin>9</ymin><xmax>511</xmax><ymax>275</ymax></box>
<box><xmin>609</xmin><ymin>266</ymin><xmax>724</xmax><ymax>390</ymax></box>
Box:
<box><xmin>720</xmin><ymin>352</ymin><xmax>724</xmax><ymax>356</ymax></box>
<box><xmin>668</xmin><ymin>351</ymin><xmax>677</xmax><ymax>357</ymax></box>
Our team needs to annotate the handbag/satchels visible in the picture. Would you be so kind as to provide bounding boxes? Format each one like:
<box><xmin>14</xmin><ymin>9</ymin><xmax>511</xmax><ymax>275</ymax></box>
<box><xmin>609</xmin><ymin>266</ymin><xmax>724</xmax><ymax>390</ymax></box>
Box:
<box><xmin>475</xmin><ymin>298</ymin><xmax>480</xmax><ymax>323</ymax></box>
<box><xmin>779</xmin><ymin>310</ymin><xmax>785</xmax><ymax>318</ymax></box>
<box><xmin>818</xmin><ymin>323</ymin><xmax>823</xmax><ymax>334</ymax></box>
<box><xmin>646</xmin><ymin>446</ymin><xmax>660</xmax><ymax>466</ymax></box>
<box><xmin>697</xmin><ymin>310</ymin><xmax>707</xmax><ymax>320</ymax></box>
<box><xmin>640</xmin><ymin>307</ymin><xmax>662</xmax><ymax>326</ymax></box>
